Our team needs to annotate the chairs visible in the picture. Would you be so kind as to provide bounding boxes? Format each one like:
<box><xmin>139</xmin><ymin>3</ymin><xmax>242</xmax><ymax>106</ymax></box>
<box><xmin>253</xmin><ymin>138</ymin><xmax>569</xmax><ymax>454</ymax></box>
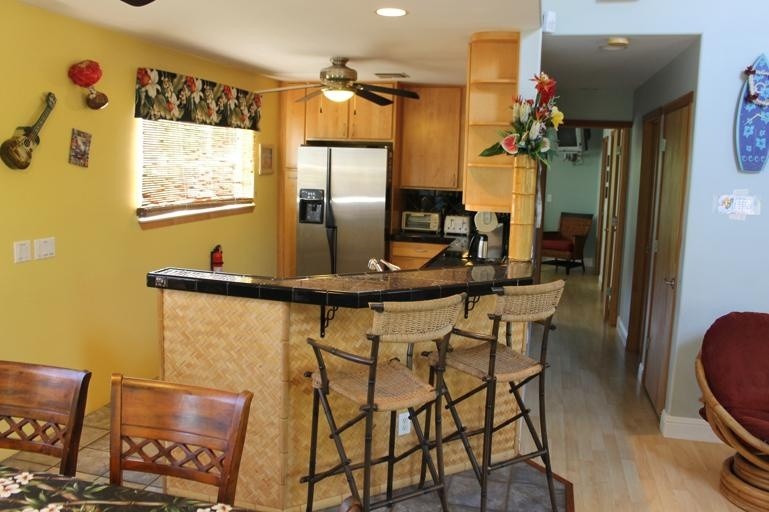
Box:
<box><xmin>542</xmin><ymin>211</ymin><xmax>594</xmax><ymax>274</ymax></box>
<box><xmin>419</xmin><ymin>278</ymin><xmax>565</xmax><ymax>512</ymax></box>
<box><xmin>300</xmin><ymin>293</ymin><xmax>464</xmax><ymax>512</ymax></box>
<box><xmin>694</xmin><ymin>310</ymin><xmax>769</xmax><ymax>512</ymax></box>
<box><xmin>0</xmin><ymin>359</ymin><xmax>254</xmax><ymax>512</ymax></box>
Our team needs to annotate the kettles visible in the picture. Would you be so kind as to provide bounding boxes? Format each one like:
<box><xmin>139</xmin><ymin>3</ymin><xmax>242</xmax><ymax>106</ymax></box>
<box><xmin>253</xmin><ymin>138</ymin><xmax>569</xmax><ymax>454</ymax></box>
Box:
<box><xmin>467</xmin><ymin>234</ymin><xmax>489</xmax><ymax>263</ymax></box>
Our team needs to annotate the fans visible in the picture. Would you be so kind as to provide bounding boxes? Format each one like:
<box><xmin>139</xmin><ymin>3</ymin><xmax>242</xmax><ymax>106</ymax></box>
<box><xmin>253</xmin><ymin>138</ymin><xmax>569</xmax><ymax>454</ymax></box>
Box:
<box><xmin>254</xmin><ymin>56</ymin><xmax>420</xmax><ymax>106</ymax></box>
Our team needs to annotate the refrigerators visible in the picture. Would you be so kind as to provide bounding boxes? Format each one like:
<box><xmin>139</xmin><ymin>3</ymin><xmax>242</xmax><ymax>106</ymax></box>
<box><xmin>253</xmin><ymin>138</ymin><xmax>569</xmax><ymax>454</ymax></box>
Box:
<box><xmin>295</xmin><ymin>143</ymin><xmax>390</xmax><ymax>275</ymax></box>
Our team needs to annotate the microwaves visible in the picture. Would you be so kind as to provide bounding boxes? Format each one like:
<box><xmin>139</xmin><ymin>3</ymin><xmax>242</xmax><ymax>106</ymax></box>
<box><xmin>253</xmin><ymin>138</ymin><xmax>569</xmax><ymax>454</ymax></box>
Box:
<box><xmin>402</xmin><ymin>211</ymin><xmax>441</xmax><ymax>235</ymax></box>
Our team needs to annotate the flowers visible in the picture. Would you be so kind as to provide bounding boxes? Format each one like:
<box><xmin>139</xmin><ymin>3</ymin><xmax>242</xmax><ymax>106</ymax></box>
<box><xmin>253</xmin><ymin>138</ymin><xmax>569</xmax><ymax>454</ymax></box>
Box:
<box><xmin>136</xmin><ymin>68</ymin><xmax>261</xmax><ymax>131</ymax></box>
<box><xmin>479</xmin><ymin>74</ymin><xmax>583</xmax><ymax>169</ymax></box>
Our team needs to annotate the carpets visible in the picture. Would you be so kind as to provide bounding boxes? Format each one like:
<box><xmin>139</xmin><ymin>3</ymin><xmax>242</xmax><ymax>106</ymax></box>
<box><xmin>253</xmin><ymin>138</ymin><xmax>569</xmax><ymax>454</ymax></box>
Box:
<box><xmin>311</xmin><ymin>454</ymin><xmax>575</xmax><ymax>512</ymax></box>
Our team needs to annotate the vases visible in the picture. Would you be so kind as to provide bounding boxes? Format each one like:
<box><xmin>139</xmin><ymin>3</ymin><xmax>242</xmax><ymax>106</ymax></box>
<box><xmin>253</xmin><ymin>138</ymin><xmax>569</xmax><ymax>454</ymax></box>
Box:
<box><xmin>508</xmin><ymin>152</ymin><xmax>538</xmax><ymax>279</ymax></box>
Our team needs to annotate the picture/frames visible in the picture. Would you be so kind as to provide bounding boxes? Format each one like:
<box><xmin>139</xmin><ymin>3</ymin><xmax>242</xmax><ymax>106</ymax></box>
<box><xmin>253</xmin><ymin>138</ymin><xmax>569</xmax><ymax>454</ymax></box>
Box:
<box><xmin>69</xmin><ymin>128</ymin><xmax>92</xmax><ymax>167</ymax></box>
<box><xmin>259</xmin><ymin>143</ymin><xmax>275</xmax><ymax>175</ymax></box>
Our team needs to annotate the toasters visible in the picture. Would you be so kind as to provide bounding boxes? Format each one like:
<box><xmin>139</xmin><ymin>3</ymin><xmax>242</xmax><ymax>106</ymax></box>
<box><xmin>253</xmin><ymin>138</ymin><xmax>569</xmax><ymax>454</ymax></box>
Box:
<box><xmin>443</xmin><ymin>216</ymin><xmax>470</xmax><ymax>239</ymax></box>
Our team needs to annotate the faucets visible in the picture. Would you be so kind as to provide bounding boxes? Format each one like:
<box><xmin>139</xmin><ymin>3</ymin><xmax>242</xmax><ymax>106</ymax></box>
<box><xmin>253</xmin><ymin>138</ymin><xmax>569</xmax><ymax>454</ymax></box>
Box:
<box><xmin>367</xmin><ymin>258</ymin><xmax>382</xmax><ymax>272</ymax></box>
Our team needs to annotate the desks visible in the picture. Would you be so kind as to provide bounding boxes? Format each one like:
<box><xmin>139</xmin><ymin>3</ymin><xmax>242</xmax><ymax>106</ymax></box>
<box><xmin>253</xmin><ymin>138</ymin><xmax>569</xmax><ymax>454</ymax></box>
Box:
<box><xmin>0</xmin><ymin>463</ymin><xmax>263</xmax><ymax>512</ymax></box>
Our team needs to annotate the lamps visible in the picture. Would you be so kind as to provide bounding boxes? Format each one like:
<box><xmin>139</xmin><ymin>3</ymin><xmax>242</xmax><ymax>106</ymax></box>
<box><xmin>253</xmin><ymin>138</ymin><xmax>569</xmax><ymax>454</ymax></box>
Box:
<box><xmin>322</xmin><ymin>89</ymin><xmax>355</xmax><ymax>102</ymax></box>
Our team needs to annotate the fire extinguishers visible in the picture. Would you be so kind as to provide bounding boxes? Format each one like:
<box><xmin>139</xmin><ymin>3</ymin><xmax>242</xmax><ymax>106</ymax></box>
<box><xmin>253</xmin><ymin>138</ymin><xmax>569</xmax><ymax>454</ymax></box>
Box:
<box><xmin>210</xmin><ymin>244</ymin><xmax>224</xmax><ymax>273</ymax></box>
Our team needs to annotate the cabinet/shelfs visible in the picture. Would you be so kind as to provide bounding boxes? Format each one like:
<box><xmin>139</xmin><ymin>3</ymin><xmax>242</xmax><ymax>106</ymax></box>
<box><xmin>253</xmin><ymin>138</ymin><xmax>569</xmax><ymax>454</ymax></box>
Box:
<box><xmin>400</xmin><ymin>86</ymin><xmax>464</xmax><ymax>191</ymax></box>
<box><xmin>390</xmin><ymin>241</ymin><xmax>448</xmax><ymax>271</ymax></box>
<box><xmin>305</xmin><ymin>83</ymin><xmax>393</xmax><ymax>143</ymax></box>
